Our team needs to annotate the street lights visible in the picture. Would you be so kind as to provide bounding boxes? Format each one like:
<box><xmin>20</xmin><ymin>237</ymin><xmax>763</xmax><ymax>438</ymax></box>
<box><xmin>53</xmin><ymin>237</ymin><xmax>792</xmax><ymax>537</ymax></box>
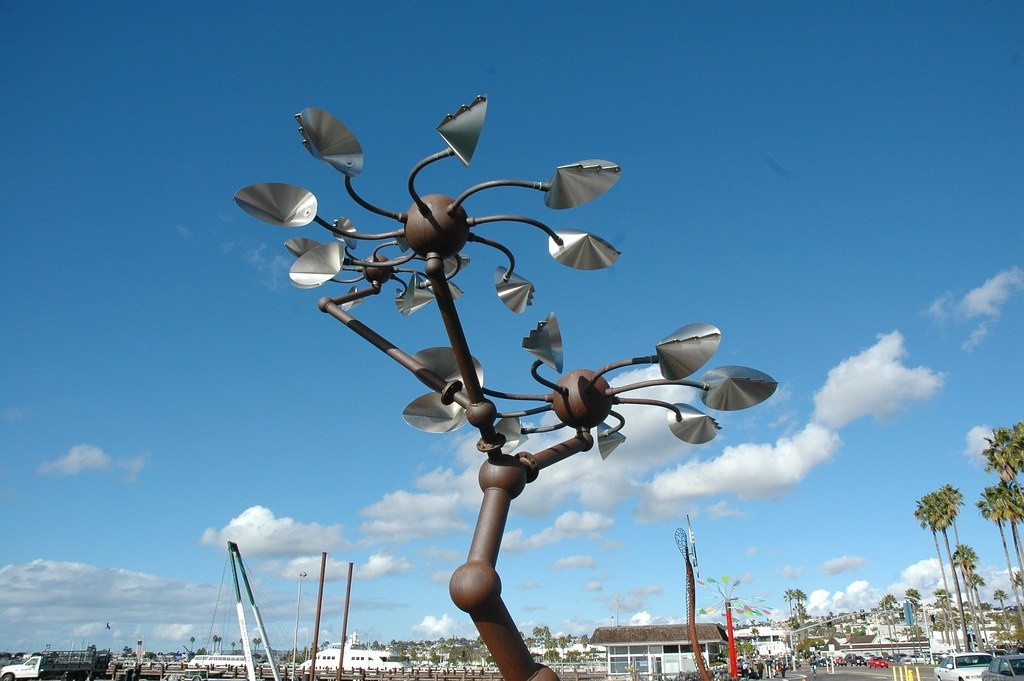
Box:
<box><xmin>290</xmin><ymin>572</ymin><xmax>307</xmax><ymax>681</ymax></box>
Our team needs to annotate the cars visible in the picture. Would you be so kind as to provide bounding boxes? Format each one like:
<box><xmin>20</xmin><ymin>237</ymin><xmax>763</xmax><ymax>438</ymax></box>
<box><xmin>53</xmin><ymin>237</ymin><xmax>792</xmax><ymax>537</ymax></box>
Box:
<box><xmin>850</xmin><ymin>656</ymin><xmax>867</xmax><ymax>667</ymax></box>
<box><xmin>808</xmin><ymin>657</ymin><xmax>848</xmax><ymax>667</ymax></box>
<box><xmin>980</xmin><ymin>653</ymin><xmax>1024</xmax><ymax>681</ymax></box>
<box><xmin>933</xmin><ymin>651</ymin><xmax>995</xmax><ymax>681</ymax></box>
<box><xmin>884</xmin><ymin>651</ymin><xmax>949</xmax><ymax>666</ymax></box>
<box><xmin>984</xmin><ymin>650</ymin><xmax>1010</xmax><ymax>659</ymax></box>
<box><xmin>866</xmin><ymin>656</ymin><xmax>889</xmax><ymax>669</ymax></box>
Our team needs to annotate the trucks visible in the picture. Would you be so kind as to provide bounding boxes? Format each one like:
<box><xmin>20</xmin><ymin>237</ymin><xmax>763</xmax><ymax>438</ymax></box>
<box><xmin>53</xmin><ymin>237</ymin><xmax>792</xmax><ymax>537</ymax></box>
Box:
<box><xmin>0</xmin><ymin>645</ymin><xmax>112</xmax><ymax>681</ymax></box>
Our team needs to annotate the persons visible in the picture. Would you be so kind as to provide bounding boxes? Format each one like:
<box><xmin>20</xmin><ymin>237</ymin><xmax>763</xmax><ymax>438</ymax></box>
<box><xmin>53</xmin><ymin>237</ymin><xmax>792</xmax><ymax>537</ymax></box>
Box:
<box><xmin>810</xmin><ymin>656</ymin><xmax>818</xmax><ymax>678</ymax></box>
<box><xmin>757</xmin><ymin>660</ymin><xmax>765</xmax><ymax>678</ymax></box>
<box><xmin>780</xmin><ymin>662</ymin><xmax>786</xmax><ymax>678</ymax></box>
